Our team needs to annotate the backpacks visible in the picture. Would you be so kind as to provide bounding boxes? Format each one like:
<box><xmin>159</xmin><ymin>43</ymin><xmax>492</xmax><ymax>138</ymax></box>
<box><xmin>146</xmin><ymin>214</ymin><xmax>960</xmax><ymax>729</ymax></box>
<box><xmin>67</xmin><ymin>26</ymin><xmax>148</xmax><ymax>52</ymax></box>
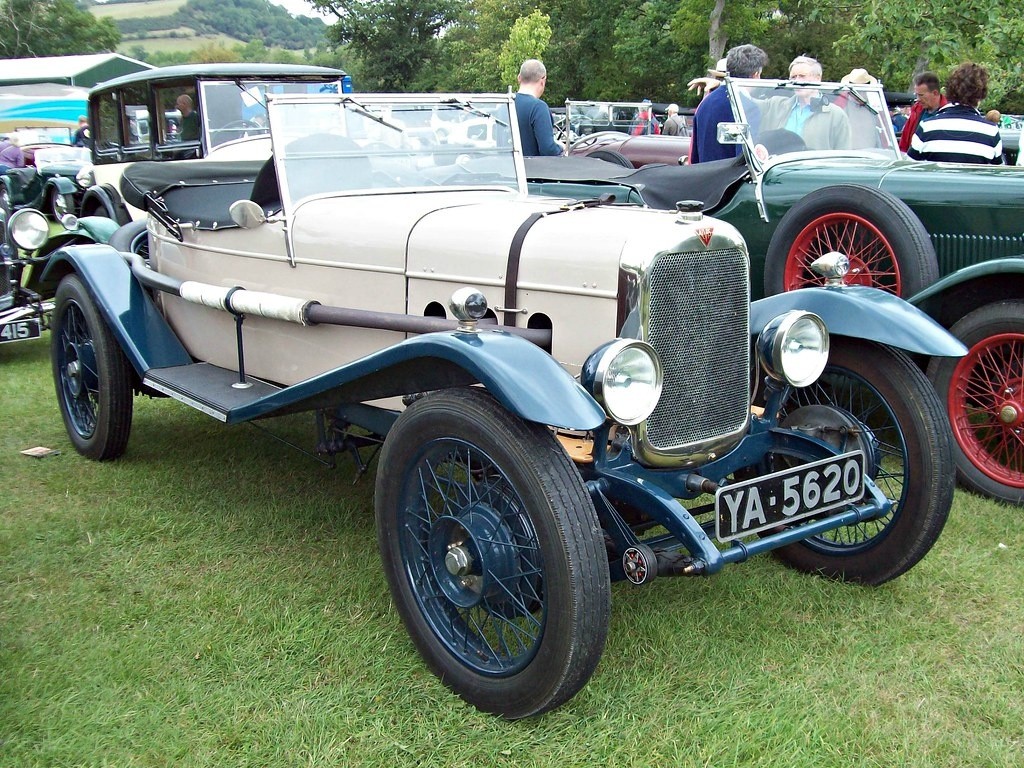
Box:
<box><xmin>670</xmin><ymin>117</ymin><xmax>689</xmax><ymax>137</ymax></box>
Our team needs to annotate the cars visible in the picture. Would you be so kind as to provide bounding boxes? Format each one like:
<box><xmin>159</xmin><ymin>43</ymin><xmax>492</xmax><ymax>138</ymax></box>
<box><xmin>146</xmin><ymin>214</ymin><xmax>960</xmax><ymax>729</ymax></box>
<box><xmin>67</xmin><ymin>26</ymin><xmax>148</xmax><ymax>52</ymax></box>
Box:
<box><xmin>0</xmin><ymin>165</ymin><xmax>134</xmax><ymax>344</ymax></box>
<box><xmin>522</xmin><ymin>79</ymin><xmax>1023</xmax><ymax>508</ymax></box>
<box><xmin>82</xmin><ymin>61</ymin><xmax>360</xmax><ymax>225</ymax></box>
<box><xmin>40</xmin><ymin>91</ymin><xmax>972</xmax><ymax>721</ymax></box>
<box><xmin>567</xmin><ymin>123</ymin><xmax>695</xmax><ymax>166</ymax></box>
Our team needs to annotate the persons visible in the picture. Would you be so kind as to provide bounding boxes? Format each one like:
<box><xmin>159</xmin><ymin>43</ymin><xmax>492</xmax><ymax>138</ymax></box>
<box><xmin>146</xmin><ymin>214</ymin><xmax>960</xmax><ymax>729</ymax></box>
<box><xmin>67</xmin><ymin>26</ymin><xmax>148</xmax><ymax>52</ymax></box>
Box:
<box><xmin>495</xmin><ymin>59</ymin><xmax>566</xmax><ymax>156</ymax></box>
<box><xmin>0</xmin><ymin>134</ymin><xmax>24</xmax><ymax>168</ymax></box>
<box><xmin>662</xmin><ymin>103</ymin><xmax>689</xmax><ymax>137</ymax></box>
<box><xmin>627</xmin><ymin>99</ymin><xmax>660</xmax><ymax>136</ymax></box>
<box><xmin>176</xmin><ymin>94</ymin><xmax>202</xmax><ymax>160</ymax></box>
<box><xmin>688</xmin><ymin>44</ymin><xmax>1008</xmax><ymax>165</ymax></box>
<box><xmin>73</xmin><ymin>115</ymin><xmax>90</xmax><ymax>144</ymax></box>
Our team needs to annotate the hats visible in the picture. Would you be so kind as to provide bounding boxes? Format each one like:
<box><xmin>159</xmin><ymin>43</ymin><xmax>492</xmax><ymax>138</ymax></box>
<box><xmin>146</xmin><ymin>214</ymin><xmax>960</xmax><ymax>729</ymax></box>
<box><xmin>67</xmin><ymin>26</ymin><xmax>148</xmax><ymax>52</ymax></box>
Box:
<box><xmin>840</xmin><ymin>68</ymin><xmax>877</xmax><ymax>85</ymax></box>
<box><xmin>665</xmin><ymin>104</ymin><xmax>679</xmax><ymax>112</ymax></box>
<box><xmin>708</xmin><ymin>58</ymin><xmax>728</xmax><ymax>77</ymax></box>
